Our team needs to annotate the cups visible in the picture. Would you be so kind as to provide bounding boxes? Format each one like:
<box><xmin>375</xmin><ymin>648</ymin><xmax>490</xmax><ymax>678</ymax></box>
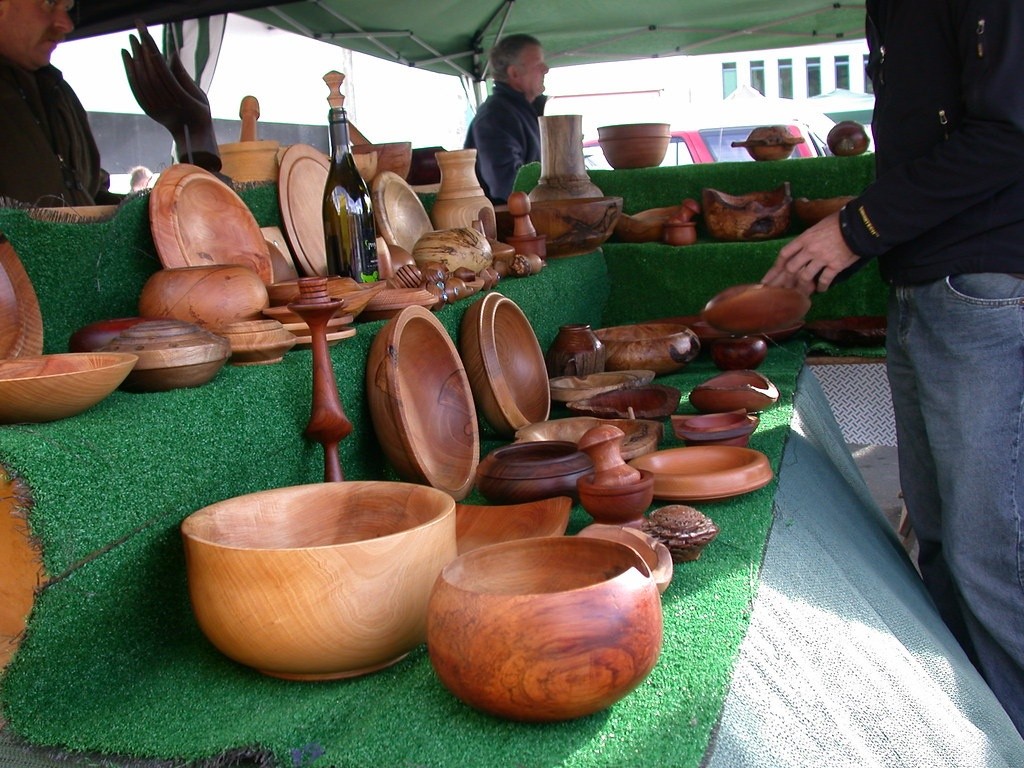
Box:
<box><xmin>662</xmin><ymin>222</ymin><xmax>697</xmax><ymax>246</ymax></box>
<box><xmin>219</xmin><ymin>140</ymin><xmax>280</xmax><ymax>183</ymax></box>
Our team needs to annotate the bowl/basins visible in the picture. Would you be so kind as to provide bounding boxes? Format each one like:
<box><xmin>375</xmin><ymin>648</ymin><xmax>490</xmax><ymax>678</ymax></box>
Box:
<box><xmin>350</xmin><ymin>142</ymin><xmax>412</xmax><ymax>181</ymax></box>
<box><xmin>179</xmin><ymin>481</ymin><xmax>456</xmax><ymax>682</ymax></box>
<box><xmin>576</xmin><ymin>469</ymin><xmax>654</xmax><ymax>531</ymax></box>
<box><xmin>460</xmin><ymin>290</ymin><xmax>551</xmax><ymax>438</ymax></box>
<box><xmin>0</xmin><ymin>352</ymin><xmax>139</xmax><ymax>422</ymax></box>
<box><xmin>492</xmin><ymin>197</ymin><xmax>622</xmax><ymax>258</ymax></box>
<box><xmin>591</xmin><ymin>324</ymin><xmax>699</xmax><ymax>373</ymax></box>
<box><xmin>426</xmin><ymin>537</ymin><xmax>661</xmax><ymax>724</ymax></box>
<box><xmin>365</xmin><ymin>304</ymin><xmax>480</xmax><ymax>502</ymax></box>
<box><xmin>796</xmin><ymin>195</ymin><xmax>858</xmax><ymax>227</ymax></box>
<box><xmin>597</xmin><ymin>123</ymin><xmax>671</xmax><ymax>169</ymax></box>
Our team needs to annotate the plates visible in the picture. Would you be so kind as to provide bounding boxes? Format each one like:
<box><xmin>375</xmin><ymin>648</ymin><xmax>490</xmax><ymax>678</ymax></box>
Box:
<box><xmin>371</xmin><ymin>171</ymin><xmax>435</xmax><ymax>255</ymax></box>
<box><xmin>640</xmin><ymin>315</ymin><xmax>806</xmax><ymax>355</ymax></box>
<box><xmin>625</xmin><ymin>444</ymin><xmax>774</xmax><ymax>504</ymax></box>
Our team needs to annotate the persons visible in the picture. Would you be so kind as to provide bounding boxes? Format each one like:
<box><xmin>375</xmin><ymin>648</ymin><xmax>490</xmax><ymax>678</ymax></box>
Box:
<box><xmin>760</xmin><ymin>0</ymin><xmax>1024</xmax><ymax>733</ymax></box>
<box><xmin>0</xmin><ymin>0</ymin><xmax>130</xmax><ymax>206</ymax></box>
<box><xmin>465</xmin><ymin>34</ymin><xmax>549</xmax><ymax>201</ymax></box>
<box><xmin>122</xmin><ymin>18</ymin><xmax>222</xmax><ymax>172</ymax></box>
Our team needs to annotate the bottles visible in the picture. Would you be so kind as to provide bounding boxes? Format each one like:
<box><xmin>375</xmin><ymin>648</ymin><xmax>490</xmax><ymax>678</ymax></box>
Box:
<box><xmin>322</xmin><ymin>107</ymin><xmax>379</xmax><ymax>285</ymax></box>
<box><xmin>711</xmin><ymin>332</ymin><xmax>766</xmax><ymax>372</ymax></box>
<box><xmin>474</xmin><ymin>442</ymin><xmax>595</xmax><ymax>510</ymax></box>
<box><xmin>432</xmin><ymin>149</ymin><xmax>497</xmax><ymax>241</ymax></box>
<box><xmin>526</xmin><ymin>114</ymin><xmax>605</xmax><ymax>209</ymax></box>
<box><xmin>545</xmin><ymin>323</ymin><xmax>605</xmax><ymax>379</ymax></box>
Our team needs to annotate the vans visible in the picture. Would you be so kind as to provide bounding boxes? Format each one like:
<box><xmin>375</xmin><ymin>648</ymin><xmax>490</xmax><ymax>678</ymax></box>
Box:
<box><xmin>579</xmin><ymin>124</ymin><xmax>822</xmax><ymax>172</ymax></box>
